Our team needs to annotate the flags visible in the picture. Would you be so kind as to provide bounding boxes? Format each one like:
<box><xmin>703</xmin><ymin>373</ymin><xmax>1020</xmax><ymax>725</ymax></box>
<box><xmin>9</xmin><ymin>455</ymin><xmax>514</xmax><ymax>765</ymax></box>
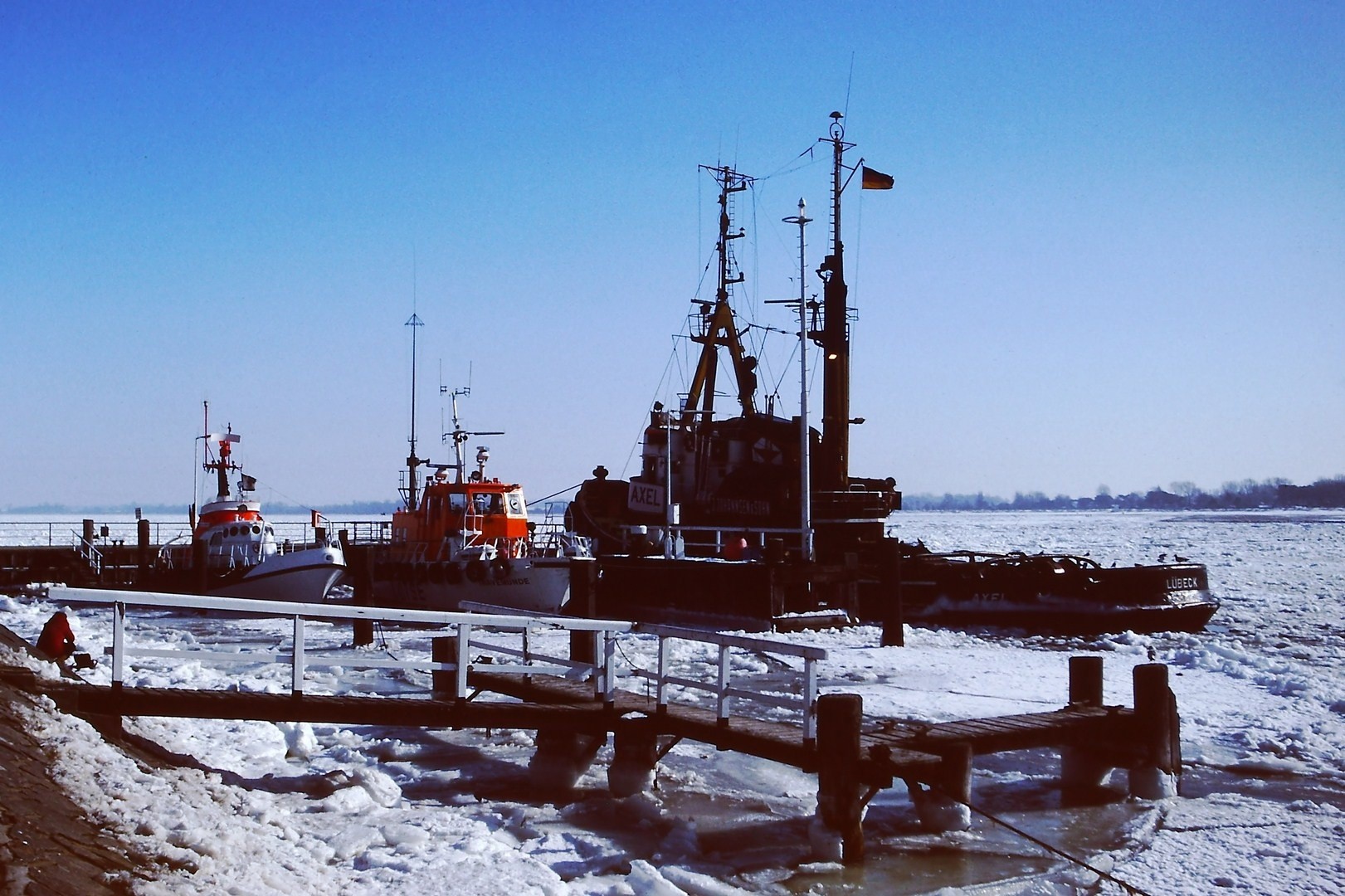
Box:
<box><xmin>862</xmin><ymin>166</ymin><xmax>894</xmax><ymax>189</ymax></box>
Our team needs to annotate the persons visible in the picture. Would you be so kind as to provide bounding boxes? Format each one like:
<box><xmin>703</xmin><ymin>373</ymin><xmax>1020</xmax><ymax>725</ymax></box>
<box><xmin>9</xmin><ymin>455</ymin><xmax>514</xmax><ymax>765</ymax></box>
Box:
<box><xmin>35</xmin><ymin>612</ymin><xmax>76</xmax><ymax>661</ymax></box>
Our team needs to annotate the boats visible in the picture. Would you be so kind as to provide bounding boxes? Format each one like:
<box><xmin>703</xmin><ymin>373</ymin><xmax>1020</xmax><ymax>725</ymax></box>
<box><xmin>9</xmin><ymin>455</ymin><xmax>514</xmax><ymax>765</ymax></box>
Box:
<box><xmin>562</xmin><ymin>87</ymin><xmax>1222</xmax><ymax>641</ymax></box>
<box><xmin>352</xmin><ymin>311</ymin><xmax>578</xmax><ymax>614</ymax></box>
<box><xmin>147</xmin><ymin>400</ymin><xmax>346</xmax><ymax>605</ymax></box>
